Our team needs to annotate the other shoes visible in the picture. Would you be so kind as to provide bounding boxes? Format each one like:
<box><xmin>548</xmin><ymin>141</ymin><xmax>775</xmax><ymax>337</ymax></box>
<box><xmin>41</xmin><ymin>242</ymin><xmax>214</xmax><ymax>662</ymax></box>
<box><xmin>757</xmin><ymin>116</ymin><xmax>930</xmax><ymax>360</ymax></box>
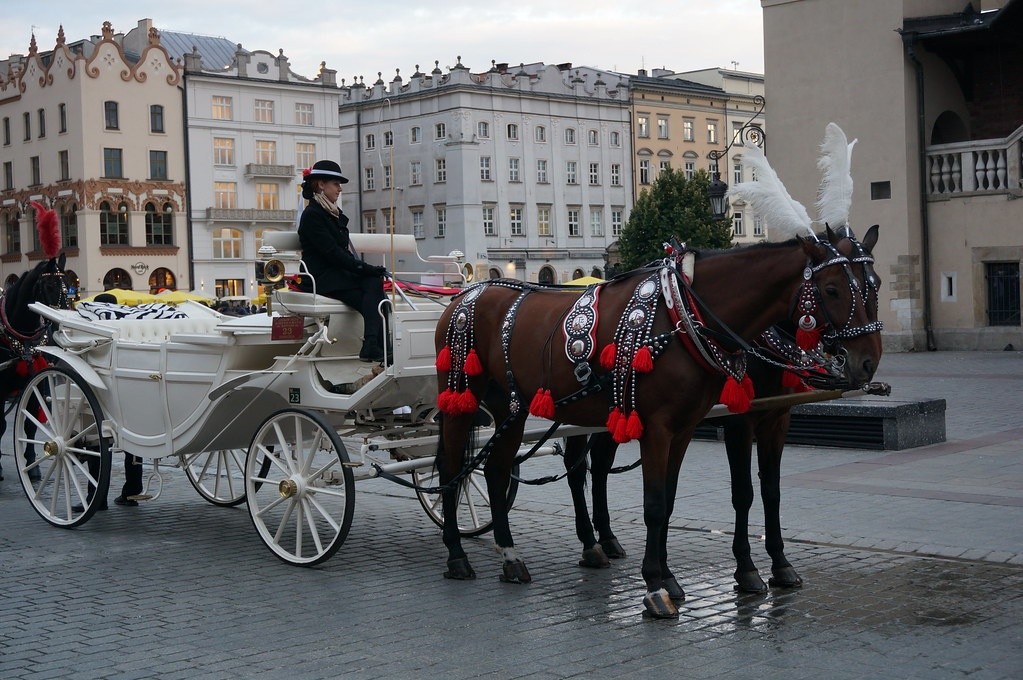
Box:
<box><xmin>359</xmin><ymin>343</ymin><xmax>393</xmax><ymax>363</ymax></box>
<box><xmin>71</xmin><ymin>497</ymin><xmax>108</xmax><ymax>511</ymax></box>
<box><xmin>114</xmin><ymin>494</ymin><xmax>138</xmax><ymax>506</ymax></box>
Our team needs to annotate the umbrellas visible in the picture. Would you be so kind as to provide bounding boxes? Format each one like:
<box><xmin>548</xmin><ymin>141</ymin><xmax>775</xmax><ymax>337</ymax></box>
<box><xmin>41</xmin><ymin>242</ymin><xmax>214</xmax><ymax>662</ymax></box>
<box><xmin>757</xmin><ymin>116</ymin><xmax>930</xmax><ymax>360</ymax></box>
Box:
<box><xmin>563</xmin><ymin>276</ymin><xmax>601</xmax><ymax>285</ymax></box>
<box><xmin>251</xmin><ymin>288</ymin><xmax>288</xmax><ymax>304</ymax></box>
<box><xmin>81</xmin><ymin>287</ymin><xmax>212</xmax><ymax>306</ymax></box>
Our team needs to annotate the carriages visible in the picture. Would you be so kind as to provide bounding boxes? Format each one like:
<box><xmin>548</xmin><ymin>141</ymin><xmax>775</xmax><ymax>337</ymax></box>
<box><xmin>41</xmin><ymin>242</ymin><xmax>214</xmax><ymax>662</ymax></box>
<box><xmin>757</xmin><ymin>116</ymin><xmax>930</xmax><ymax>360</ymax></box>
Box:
<box><xmin>11</xmin><ymin>121</ymin><xmax>892</xmax><ymax>621</ymax></box>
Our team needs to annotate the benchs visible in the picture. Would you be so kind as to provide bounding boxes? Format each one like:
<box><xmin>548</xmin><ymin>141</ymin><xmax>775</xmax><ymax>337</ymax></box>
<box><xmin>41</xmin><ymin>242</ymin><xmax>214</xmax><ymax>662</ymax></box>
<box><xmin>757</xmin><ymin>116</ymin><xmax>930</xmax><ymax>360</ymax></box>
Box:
<box><xmin>255</xmin><ymin>231</ymin><xmax>474</xmax><ymax>356</ymax></box>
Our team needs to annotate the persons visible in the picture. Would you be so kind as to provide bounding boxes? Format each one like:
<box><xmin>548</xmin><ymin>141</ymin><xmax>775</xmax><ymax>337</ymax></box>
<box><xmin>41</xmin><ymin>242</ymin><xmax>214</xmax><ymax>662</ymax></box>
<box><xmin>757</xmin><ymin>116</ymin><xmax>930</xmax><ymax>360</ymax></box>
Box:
<box><xmin>245</xmin><ymin>301</ymin><xmax>250</xmax><ymax>314</ymax></box>
<box><xmin>69</xmin><ymin>294</ymin><xmax>143</xmax><ymax>509</ymax></box>
<box><xmin>299</xmin><ymin>160</ymin><xmax>393</xmax><ymax>363</ymax></box>
<box><xmin>235</xmin><ymin>305</ymin><xmax>240</xmax><ymax>312</ymax></box>
<box><xmin>259</xmin><ymin>303</ymin><xmax>266</xmax><ymax>312</ymax></box>
<box><xmin>251</xmin><ymin>305</ymin><xmax>257</xmax><ymax>314</ymax></box>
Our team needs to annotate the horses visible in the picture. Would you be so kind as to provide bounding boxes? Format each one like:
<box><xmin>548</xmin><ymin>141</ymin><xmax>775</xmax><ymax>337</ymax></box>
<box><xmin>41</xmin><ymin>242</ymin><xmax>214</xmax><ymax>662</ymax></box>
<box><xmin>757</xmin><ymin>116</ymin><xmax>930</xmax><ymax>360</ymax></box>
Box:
<box><xmin>0</xmin><ymin>252</ymin><xmax>69</xmax><ymax>482</ymax></box>
<box><xmin>431</xmin><ymin>234</ymin><xmax>881</xmax><ymax>618</ymax></box>
<box><xmin>564</xmin><ymin>222</ymin><xmax>882</xmax><ymax>599</ymax></box>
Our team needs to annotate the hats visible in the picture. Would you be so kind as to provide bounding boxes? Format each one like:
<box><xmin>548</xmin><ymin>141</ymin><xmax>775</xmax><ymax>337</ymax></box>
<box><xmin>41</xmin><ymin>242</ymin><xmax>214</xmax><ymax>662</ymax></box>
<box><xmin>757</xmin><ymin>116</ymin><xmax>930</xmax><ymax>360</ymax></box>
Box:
<box><xmin>303</xmin><ymin>160</ymin><xmax>349</xmax><ymax>183</ymax></box>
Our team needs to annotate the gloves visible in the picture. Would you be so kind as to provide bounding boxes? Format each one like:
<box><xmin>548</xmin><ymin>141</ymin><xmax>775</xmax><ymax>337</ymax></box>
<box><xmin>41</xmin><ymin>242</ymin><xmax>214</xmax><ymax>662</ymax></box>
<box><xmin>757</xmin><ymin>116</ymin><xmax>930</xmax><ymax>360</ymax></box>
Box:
<box><xmin>367</xmin><ymin>264</ymin><xmax>386</xmax><ymax>278</ymax></box>
